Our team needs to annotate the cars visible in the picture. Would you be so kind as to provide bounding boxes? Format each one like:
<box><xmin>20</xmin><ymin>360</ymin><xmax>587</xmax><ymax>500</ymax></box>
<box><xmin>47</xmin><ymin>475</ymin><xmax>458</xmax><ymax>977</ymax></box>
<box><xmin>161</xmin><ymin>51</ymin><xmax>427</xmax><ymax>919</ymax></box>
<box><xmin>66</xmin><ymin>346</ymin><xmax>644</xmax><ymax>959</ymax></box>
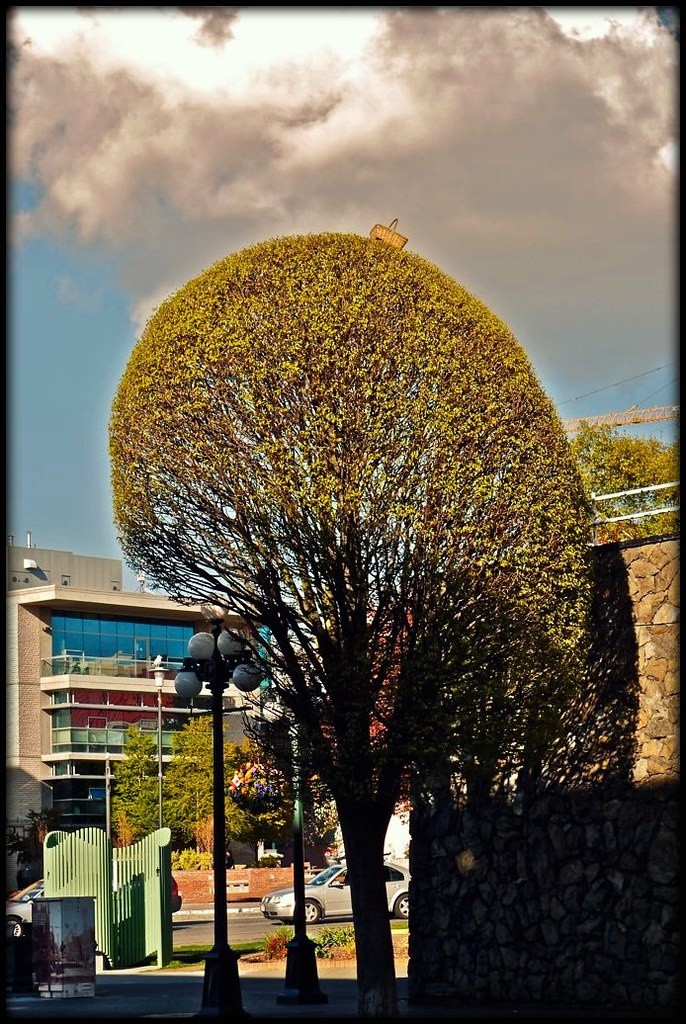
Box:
<box><xmin>6</xmin><ymin>869</ymin><xmax>183</xmax><ymax>938</ymax></box>
<box><xmin>258</xmin><ymin>859</ymin><xmax>411</xmax><ymax>926</ymax></box>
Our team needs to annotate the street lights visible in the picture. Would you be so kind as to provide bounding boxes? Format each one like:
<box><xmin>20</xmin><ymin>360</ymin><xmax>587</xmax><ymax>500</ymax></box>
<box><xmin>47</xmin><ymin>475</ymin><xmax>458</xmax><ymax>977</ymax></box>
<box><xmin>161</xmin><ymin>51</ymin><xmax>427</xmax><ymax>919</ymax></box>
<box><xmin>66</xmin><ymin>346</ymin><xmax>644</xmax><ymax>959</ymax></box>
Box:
<box><xmin>173</xmin><ymin>590</ymin><xmax>265</xmax><ymax>1024</ymax></box>
